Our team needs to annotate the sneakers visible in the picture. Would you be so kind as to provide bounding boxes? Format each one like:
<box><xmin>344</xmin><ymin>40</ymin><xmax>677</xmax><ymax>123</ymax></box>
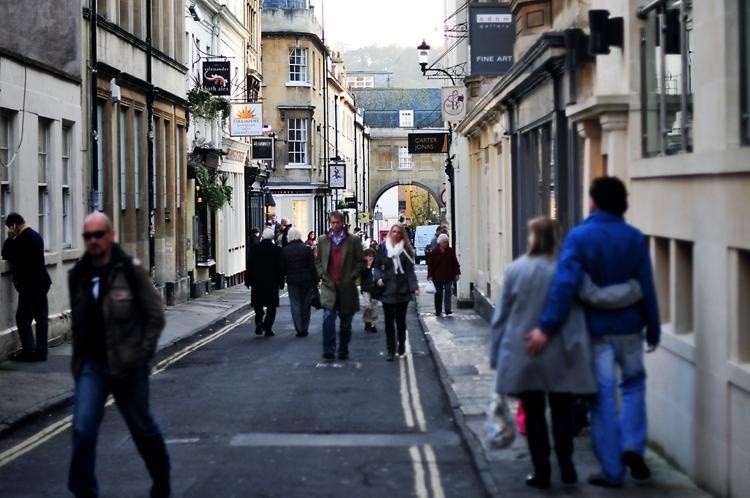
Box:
<box><xmin>253</xmin><ymin>326</ymin><xmax>276</xmax><ymax>339</ymax></box>
<box><xmin>619</xmin><ymin>449</ymin><xmax>651</xmax><ymax>481</ymax></box>
<box><xmin>364</xmin><ymin>321</ymin><xmax>377</xmax><ymax>333</ymax></box>
<box><xmin>434</xmin><ymin>311</ymin><xmax>453</xmax><ymax>317</ymax></box>
<box><xmin>525</xmin><ymin>472</ymin><xmax>552</xmax><ymax>490</ymax></box>
<box><xmin>587</xmin><ymin>473</ymin><xmax>625</xmax><ymax>489</ymax></box>
<box><xmin>293</xmin><ymin>329</ymin><xmax>309</xmax><ymax>337</ymax></box>
<box><xmin>560</xmin><ymin>471</ymin><xmax>578</xmax><ymax>485</ymax></box>
<box><xmin>321</xmin><ymin>351</ymin><xmax>349</xmax><ymax>363</ymax></box>
<box><xmin>7</xmin><ymin>347</ymin><xmax>48</xmax><ymax>363</ymax></box>
<box><xmin>384</xmin><ymin>352</ymin><xmax>405</xmax><ymax>361</ymax></box>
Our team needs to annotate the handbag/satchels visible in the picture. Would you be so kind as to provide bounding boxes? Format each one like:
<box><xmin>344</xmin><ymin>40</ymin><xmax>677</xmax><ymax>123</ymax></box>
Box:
<box><xmin>309</xmin><ymin>286</ymin><xmax>321</xmax><ymax>310</ymax></box>
<box><xmin>482</xmin><ymin>371</ymin><xmax>517</xmax><ymax>451</ymax></box>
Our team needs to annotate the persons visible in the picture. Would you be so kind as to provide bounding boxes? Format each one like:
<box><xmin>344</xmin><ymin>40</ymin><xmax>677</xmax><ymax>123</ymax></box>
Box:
<box><xmin>65</xmin><ymin>212</ymin><xmax>172</xmax><ymax>497</ymax></box>
<box><xmin>2</xmin><ymin>212</ymin><xmax>53</xmax><ymax>362</ymax></box>
<box><xmin>524</xmin><ymin>176</ymin><xmax>661</xmax><ymax>488</ymax></box>
<box><xmin>487</xmin><ymin>214</ymin><xmax>644</xmax><ymax>489</ymax></box>
<box><xmin>246</xmin><ymin>211</ymin><xmax>462</xmax><ymax>362</ymax></box>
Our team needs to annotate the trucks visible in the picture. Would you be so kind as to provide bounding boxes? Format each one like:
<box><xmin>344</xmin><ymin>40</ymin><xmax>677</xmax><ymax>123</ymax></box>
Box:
<box><xmin>414</xmin><ymin>224</ymin><xmax>440</xmax><ymax>264</ymax></box>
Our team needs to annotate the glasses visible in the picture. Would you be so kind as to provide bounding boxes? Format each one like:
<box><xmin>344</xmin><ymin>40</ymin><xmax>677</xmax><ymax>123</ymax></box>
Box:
<box><xmin>80</xmin><ymin>228</ymin><xmax>107</xmax><ymax>240</ymax></box>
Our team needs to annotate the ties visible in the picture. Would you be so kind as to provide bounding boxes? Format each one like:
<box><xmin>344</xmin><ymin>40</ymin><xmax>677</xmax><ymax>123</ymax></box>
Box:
<box><xmin>91</xmin><ymin>277</ymin><xmax>101</xmax><ymax>301</ymax></box>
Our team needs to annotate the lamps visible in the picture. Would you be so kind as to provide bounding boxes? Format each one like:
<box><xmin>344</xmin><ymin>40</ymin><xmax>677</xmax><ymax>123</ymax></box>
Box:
<box><xmin>417</xmin><ymin>40</ymin><xmax>456</xmax><ymax>87</ymax></box>
